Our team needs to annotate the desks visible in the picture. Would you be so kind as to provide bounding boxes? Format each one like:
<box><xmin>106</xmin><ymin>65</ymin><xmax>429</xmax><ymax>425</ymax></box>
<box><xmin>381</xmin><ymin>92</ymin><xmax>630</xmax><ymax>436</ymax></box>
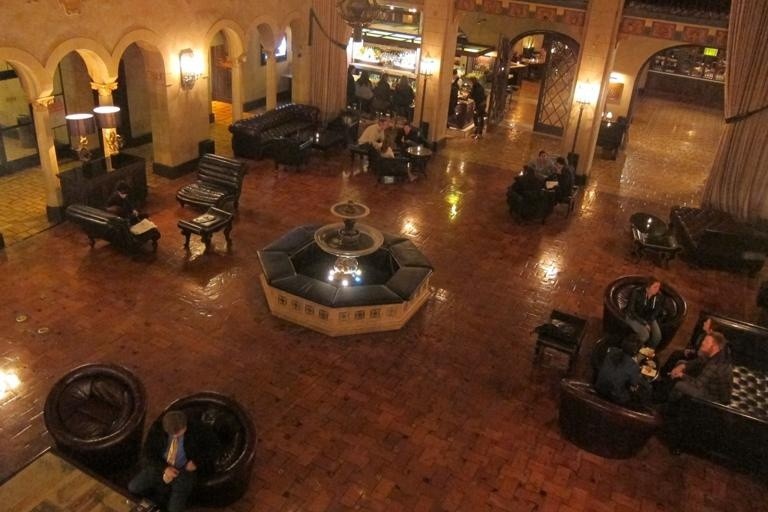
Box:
<box><xmin>0</xmin><ymin>446</ymin><xmax>161</xmax><ymax>512</ymax></box>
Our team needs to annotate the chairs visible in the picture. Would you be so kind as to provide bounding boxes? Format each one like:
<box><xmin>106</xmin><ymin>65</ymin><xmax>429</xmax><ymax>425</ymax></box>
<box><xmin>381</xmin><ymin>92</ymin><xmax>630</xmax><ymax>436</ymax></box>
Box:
<box><xmin>603</xmin><ymin>275</ymin><xmax>687</xmax><ymax>353</ymax></box>
<box><xmin>369</xmin><ymin>147</ymin><xmax>402</xmax><ymax>183</ymax></box>
<box><xmin>509</xmin><ymin>191</ymin><xmax>549</xmax><ymax>225</ymax></box>
<box><xmin>140</xmin><ymin>390</ymin><xmax>256</xmax><ymax>508</ymax></box>
<box><xmin>267</xmin><ymin>138</ymin><xmax>307</xmax><ymax>172</ymax></box>
<box><xmin>632</xmin><ymin>236</ymin><xmax>682</xmax><ymax>268</ymax></box>
<box><xmin>559</xmin><ymin>377</ymin><xmax>663</xmax><ymax>460</ymax></box>
<box><xmin>44</xmin><ymin>362</ymin><xmax>146</xmax><ymax>475</ymax></box>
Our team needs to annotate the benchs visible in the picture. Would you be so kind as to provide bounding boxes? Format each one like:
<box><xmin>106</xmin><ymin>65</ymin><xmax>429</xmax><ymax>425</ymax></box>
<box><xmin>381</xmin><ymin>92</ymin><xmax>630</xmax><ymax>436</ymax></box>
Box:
<box><xmin>669</xmin><ymin>310</ymin><xmax>768</xmax><ymax>478</ymax></box>
<box><xmin>670</xmin><ymin>205</ymin><xmax>765</xmax><ymax>278</ymax></box>
<box><xmin>553</xmin><ymin>186</ymin><xmax>580</xmax><ymax>217</ymax></box>
<box><xmin>66</xmin><ymin>203</ymin><xmax>161</xmax><ymax>259</ymax></box>
<box><xmin>255</xmin><ymin>224</ymin><xmax>434</xmax><ymax>337</ymax></box>
<box><xmin>176</xmin><ymin>153</ymin><xmax>250</xmax><ymax>209</ymax></box>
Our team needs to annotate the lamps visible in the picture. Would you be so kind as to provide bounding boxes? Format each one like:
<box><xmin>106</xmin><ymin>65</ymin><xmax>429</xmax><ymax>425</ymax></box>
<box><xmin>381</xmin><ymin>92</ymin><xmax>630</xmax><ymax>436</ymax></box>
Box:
<box><xmin>567</xmin><ymin>82</ymin><xmax>594</xmax><ymax>170</ymax></box>
<box><xmin>65</xmin><ymin>114</ymin><xmax>98</xmax><ymax>169</ymax></box>
<box><xmin>419</xmin><ymin>58</ymin><xmax>432</xmax><ymax>141</ymax></box>
<box><xmin>93</xmin><ymin>106</ymin><xmax>126</xmax><ymax>159</ymax></box>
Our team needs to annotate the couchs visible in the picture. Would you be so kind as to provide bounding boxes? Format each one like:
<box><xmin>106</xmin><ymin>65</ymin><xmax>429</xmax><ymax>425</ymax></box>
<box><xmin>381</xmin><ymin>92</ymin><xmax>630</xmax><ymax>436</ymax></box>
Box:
<box><xmin>229</xmin><ymin>104</ymin><xmax>322</xmax><ymax>160</ymax></box>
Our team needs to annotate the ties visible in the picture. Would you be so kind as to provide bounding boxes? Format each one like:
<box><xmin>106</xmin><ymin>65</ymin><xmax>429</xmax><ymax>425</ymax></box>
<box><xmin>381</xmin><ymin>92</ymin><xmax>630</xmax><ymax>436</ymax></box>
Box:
<box><xmin>166</xmin><ymin>439</ymin><xmax>178</xmax><ymax>466</ymax></box>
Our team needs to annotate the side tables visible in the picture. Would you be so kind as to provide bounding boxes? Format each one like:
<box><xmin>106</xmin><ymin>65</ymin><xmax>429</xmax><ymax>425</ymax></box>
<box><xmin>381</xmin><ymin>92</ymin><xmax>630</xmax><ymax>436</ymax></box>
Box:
<box><xmin>406</xmin><ymin>146</ymin><xmax>432</xmax><ymax>179</ymax></box>
<box><xmin>177</xmin><ymin>207</ymin><xmax>235</xmax><ymax>249</ymax></box>
<box><xmin>533</xmin><ymin>309</ymin><xmax>587</xmax><ymax>379</ymax></box>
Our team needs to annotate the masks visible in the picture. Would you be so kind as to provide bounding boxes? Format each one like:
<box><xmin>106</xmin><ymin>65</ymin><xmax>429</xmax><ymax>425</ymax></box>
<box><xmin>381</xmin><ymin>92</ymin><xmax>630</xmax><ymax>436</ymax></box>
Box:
<box><xmin>119</xmin><ymin>193</ymin><xmax>127</xmax><ymax>198</ymax></box>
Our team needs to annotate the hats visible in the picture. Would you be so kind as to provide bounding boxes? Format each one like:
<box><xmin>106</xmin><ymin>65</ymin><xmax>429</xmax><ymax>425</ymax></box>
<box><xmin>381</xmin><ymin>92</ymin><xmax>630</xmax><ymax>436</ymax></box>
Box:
<box><xmin>117</xmin><ymin>183</ymin><xmax>132</xmax><ymax>193</ymax></box>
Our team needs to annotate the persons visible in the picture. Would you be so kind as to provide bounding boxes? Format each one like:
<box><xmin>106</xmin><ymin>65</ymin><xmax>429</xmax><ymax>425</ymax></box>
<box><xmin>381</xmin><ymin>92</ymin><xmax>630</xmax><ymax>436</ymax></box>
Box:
<box><xmin>447</xmin><ymin>68</ymin><xmax>463</xmax><ymax>129</ymax></box>
<box><xmin>592</xmin><ymin>334</ymin><xmax>653</xmax><ymax>410</ymax></box>
<box><xmin>543</xmin><ymin>156</ymin><xmax>573</xmax><ymax>198</ymax></box>
<box><xmin>539</xmin><ymin>48</ymin><xmax>547</xmax><ymax>62</ymax></box>
<box><xmin>514</xmin><ymin>166</ymin><xmax>540</xmax><ymax>190</ymax></box>
<box><xmin>659</xmin><ymin>318</ymin><xmax>720</xmax><ymax>380</ymax></box>
<box><xmin>355</xmin><ymin>117</ymin><xmax>436</xmax><ymax>183</ymax></box>
<box><xmin>104</xmin><ymin>180</ymin><xmax>152</xmax><ymax>226</ymax></box>
<box><xmin>127</xmin><ymin>410</ymin><xmax>224</xmax><ymax>511</ymax></box>
<box><xmin>466</xmin><ymin>76</ymin><xmax>487</xmax><ymax>139</ymax></box>
<box><xmin>373</xmin><ymin>73</ymin><xmax>393</xmax><ymax>120</ymax></box>
<box><xmin>347</xmin><ymin>64</ymin><xmax>355</xmax><ymax>106</ymax></box>
<box><xmin>645</xmin><ymin>330</ymin><xmax>734</xmax><ymax>437</ymax></box>
<box><xmin>511</xmin><ymin>51</ymin><xmax>521</xmax><ymax>64</ymax></box>
<box><xmin>526</xmin><ymin>150</ymin><xmax>557</xmax><ymax>181</ymax></box>
<box><xmin>354</xmin><ymin>70</ymin><xmax>375</xmax><ymax>114</ymax></box>
<box><xmin>624</xmin><ymin>274</ymin><xmax>663</xmax><ymax>352</ymax></box>
<box><xmin>392</xmin><ymin>75</ymin><xmax>414</xmax><ymax>118</ymax></box>
